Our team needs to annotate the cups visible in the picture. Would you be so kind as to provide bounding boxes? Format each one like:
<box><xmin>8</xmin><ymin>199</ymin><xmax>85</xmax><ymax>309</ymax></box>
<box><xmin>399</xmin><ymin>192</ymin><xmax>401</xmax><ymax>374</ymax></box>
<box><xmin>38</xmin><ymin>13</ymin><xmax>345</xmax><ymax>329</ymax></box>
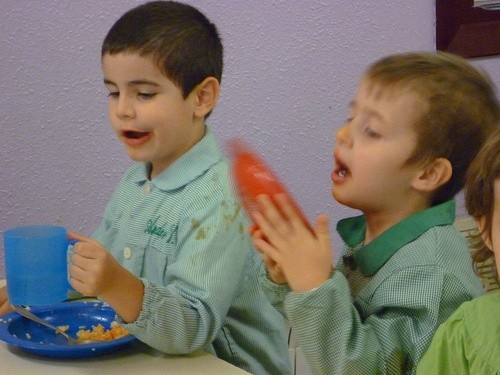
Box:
<box><xmin>3</xmin><ymin>224</ymin><xmax>79</xmax><ymax>306</ymax></box>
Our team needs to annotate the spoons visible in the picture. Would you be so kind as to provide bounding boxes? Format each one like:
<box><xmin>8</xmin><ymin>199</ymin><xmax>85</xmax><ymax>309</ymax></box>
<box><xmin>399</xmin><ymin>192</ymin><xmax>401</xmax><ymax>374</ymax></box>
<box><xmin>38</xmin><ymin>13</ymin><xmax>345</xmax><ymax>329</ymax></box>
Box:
<box><xmin>10</xmin><ymin>304</ymin><xmax>101</xmax><ymax>344</ymax></box>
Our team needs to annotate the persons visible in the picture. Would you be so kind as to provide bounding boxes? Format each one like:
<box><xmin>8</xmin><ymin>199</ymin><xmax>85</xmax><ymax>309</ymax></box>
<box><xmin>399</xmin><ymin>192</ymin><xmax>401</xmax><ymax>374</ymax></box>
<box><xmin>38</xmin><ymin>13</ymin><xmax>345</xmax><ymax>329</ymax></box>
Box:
<box><xmin>418</xmin><ymin>127</ymin><xmax>500</xmax><ymax>375</ymax></box>
<box><xmin>255</xmin><ymin>51</ymin><xmax>485</xmax><ymax>375</ymax></box>
<box><xmin>0</xmin><ymin>0</ymin><xmax>296</xmax><ymax>375</ymax></box>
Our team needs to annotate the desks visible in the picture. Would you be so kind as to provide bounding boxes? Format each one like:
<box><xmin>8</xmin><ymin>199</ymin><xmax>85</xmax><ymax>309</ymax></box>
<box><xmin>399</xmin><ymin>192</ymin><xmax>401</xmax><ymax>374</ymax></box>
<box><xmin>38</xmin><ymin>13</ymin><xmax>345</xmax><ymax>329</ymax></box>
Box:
<box><xmin>0</xmin><ymin>278</ymin><xmax>251</xmax><ymax>375</ymax></box>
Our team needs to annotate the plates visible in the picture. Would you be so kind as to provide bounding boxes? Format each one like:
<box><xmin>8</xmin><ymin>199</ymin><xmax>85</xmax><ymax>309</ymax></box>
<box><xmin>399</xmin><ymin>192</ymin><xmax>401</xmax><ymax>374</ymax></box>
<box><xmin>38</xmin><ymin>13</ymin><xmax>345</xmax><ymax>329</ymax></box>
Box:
<box><xmin>229</xmin><ymin>137</ymin><xmax>318</xmax><ymax>252</ymax></box>
<box><xmin>0</xmin><ymin>301</ymin><xmax>137</xmax><ymax>359</ymax></box>
